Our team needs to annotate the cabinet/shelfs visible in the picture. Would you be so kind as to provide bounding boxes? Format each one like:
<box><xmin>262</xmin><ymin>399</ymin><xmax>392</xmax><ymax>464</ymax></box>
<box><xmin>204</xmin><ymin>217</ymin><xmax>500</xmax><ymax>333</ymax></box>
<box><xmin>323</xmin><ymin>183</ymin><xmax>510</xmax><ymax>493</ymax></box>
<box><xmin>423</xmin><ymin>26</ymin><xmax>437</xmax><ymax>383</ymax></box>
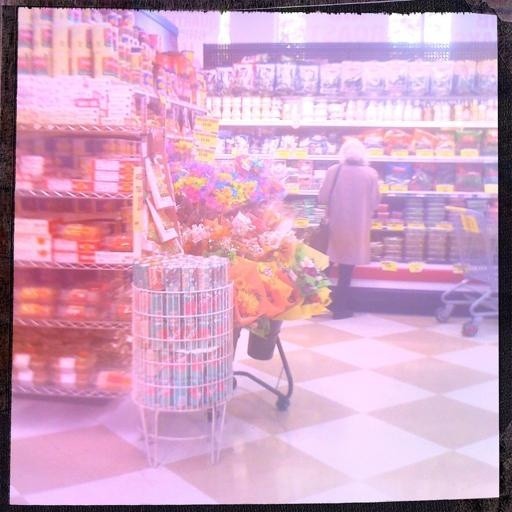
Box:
<box><xmin>13</xmin><ymin>44</ymin><xmax>501</xmax><ymax>400</ymax></box>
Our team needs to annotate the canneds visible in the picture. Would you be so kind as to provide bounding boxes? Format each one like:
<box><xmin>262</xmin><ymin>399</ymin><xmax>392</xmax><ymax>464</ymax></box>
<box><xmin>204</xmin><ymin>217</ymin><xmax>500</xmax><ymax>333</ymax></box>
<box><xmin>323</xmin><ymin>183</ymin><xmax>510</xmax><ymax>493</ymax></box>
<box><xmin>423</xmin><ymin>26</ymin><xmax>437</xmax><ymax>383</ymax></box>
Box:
<box><xmin>18</xmin><ymin>6</ymin><xmax>143</xmax><ymax>86</ymax></box>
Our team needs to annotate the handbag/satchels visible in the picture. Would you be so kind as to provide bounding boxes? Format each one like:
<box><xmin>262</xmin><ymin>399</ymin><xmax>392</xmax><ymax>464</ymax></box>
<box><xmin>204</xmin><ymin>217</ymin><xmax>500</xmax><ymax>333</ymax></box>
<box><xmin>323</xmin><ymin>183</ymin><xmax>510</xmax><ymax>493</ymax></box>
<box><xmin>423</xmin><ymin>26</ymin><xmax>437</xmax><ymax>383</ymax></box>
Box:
<box><xmin>313</xmin><ymin>218</ymin><xmax>330</xmax><ymax>254</ymax></box>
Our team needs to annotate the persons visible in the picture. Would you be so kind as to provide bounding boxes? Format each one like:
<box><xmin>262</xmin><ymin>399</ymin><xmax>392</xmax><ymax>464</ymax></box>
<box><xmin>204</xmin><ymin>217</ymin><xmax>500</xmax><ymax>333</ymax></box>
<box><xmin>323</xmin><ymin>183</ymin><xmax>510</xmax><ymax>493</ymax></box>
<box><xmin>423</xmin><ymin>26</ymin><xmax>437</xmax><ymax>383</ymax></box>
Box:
<box><xmin>316</xmin><ymin>137</ymin><xmax>382</xmax><ymax>320</ymax></box>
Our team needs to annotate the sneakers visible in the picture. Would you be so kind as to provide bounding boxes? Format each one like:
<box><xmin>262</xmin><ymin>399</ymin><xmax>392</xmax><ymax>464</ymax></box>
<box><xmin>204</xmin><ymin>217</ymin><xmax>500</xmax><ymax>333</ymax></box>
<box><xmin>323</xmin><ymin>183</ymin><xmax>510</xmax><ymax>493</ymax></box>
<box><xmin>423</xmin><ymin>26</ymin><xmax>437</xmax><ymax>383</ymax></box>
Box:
<box><xmin>333</xmin><ymin>309</ymin><xmax>353</xmax><ymax>319</ymax></box>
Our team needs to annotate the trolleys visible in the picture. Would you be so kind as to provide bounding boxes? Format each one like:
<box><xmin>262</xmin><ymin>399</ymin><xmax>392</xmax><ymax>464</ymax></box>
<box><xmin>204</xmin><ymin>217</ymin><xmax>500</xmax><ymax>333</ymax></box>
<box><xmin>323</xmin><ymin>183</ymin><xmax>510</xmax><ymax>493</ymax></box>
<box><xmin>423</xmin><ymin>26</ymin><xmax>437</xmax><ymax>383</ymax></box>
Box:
<box><xmin>430</xmin><ymin>203</ymin><xmax>499</xmax><ymax>339</ymax></box>
